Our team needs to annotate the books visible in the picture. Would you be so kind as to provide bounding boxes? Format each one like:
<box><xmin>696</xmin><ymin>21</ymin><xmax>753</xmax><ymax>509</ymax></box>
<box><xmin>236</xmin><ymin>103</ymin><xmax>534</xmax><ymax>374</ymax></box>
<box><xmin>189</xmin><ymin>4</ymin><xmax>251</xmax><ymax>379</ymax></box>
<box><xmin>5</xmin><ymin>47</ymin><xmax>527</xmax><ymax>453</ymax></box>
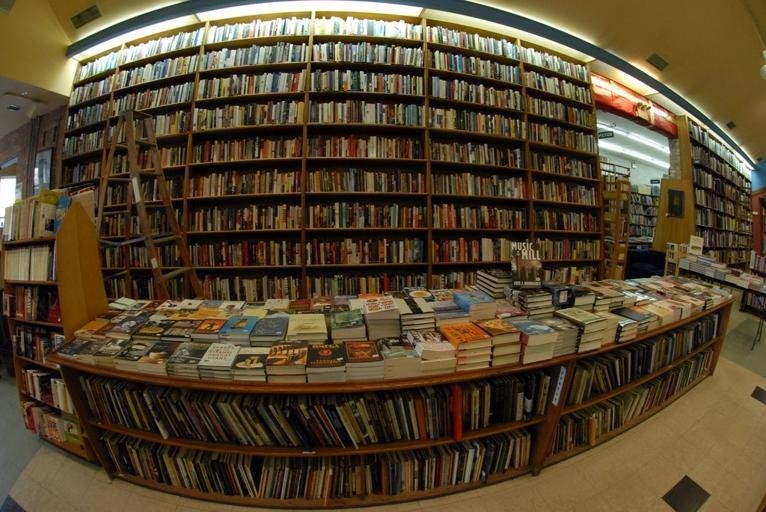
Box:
<box><xmin>667</xmin><ymin>120</ymin><xmax>765</xmax><ymax>294</ymax></box>
<box><xmin>2</xmin><ymin>190</ymin><xmax>88</xmax><ymax>459</ymax></box>
<box><xmin>58</xmin><ymin>277</ymin><xmax>733</xmax><ymax>499</ymax></box>
<box><xmin>602</xmin><ymin>175</ymin><xmax>660</xmax><ymax>279</ymax></box>
<box><xmin>60</xmin><ymin>15</ymin><xmax>603</xmax><ymax>301</ymax></box>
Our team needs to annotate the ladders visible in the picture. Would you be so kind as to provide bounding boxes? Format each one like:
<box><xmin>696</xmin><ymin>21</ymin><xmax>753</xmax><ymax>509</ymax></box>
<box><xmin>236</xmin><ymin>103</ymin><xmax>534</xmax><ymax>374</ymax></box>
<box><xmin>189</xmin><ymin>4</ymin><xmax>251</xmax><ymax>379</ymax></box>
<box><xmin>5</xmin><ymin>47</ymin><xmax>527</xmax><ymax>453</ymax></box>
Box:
<box><xmin>97</xmin><ymin>109</ymin><xmax>204</xmax><ymax>300</ymax></box>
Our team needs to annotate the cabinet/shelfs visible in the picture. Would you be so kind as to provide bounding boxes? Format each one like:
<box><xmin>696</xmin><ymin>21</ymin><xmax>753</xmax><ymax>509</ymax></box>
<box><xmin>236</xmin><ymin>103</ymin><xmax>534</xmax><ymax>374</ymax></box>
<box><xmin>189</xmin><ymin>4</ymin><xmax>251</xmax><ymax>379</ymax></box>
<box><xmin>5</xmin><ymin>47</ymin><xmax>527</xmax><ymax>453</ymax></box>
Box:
<box><xmin>0</xmin><ymin>11</ymin><xmax>765</xmax><ymax>509</ymax></box>
